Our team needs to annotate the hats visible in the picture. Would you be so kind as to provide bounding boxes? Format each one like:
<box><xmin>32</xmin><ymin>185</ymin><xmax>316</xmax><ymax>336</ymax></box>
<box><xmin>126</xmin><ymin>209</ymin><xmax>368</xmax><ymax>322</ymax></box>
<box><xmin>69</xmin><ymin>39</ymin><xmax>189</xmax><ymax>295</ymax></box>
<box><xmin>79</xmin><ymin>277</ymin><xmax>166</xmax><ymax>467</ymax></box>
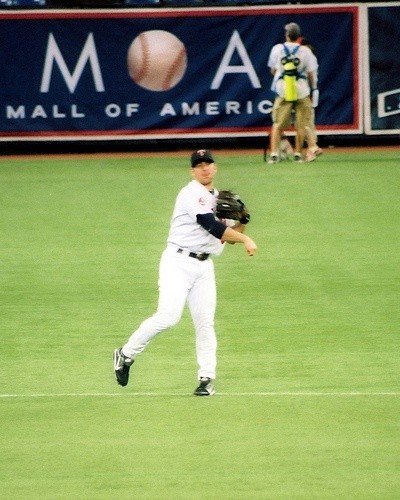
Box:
<box><xmin>285</xmin><ymin>23</ymin><xmax>300</xmax><ymax>35</ymax></box>
<box><xmin>191</xmin><ymin>149</ymin><xmax>214</xmax><ymax>167</ymax></box>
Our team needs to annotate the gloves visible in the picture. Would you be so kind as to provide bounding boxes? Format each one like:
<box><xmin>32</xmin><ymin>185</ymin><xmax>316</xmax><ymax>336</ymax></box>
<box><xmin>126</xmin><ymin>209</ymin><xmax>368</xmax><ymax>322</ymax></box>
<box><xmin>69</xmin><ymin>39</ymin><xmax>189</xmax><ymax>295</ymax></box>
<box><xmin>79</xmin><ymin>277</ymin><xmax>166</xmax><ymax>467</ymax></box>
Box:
<box><xmin>311</xmin><ymin>88</ymin><xmax>319</xmax><ymax>108</ymax></box>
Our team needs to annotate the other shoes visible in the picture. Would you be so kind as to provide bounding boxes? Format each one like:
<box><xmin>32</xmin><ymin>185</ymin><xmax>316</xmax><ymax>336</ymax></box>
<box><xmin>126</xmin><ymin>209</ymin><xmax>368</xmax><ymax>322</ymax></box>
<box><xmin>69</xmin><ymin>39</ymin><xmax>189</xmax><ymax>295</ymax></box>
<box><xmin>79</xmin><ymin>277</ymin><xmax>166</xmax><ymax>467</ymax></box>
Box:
<box><xmin>293</xmin><ymin>152</ymin><xmax>304</xmax><ymax>163</ymax></box>
<box><xmin>269</xmin><ymin>152</ymin><xmax>280</xmax><ymax>163</ymax></box>
<box><xmin>304</xmin><ymin>148</ymin><xmax>322</xmax><ymax>162</ymax></box>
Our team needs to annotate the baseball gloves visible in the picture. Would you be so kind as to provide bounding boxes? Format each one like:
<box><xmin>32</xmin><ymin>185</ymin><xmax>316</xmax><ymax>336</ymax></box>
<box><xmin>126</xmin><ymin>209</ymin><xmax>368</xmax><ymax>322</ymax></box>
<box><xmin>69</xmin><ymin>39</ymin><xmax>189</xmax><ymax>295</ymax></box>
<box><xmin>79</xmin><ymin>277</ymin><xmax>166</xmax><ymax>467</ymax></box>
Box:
<box><xmin>216</xmin><ymin>191</ymin><xmax>250</xmax><ymax>224</ymax></box>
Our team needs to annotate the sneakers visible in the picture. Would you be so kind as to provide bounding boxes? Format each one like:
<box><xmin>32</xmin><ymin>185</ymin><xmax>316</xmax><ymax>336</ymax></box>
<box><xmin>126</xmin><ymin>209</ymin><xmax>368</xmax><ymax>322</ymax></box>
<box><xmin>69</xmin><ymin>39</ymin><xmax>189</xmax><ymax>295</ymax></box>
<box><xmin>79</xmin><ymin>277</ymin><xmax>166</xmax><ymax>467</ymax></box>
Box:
<box><xmin>193</xmin><ymin>377</ymin><xmax>216</xmax><ymax>397</ymax></box>
<box><xmin>113</xmin><ymin>347</ymin><xmax>135</xmax><ymax>387</ymax></box>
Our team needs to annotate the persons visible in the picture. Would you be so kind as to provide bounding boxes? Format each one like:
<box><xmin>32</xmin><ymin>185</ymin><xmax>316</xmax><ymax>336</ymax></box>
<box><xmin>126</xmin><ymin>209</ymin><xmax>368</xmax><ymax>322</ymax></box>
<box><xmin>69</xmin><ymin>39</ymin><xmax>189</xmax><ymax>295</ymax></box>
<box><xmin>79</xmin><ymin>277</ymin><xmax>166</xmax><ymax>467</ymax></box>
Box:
<box><xmin>266</xmin><ymin>23</ymin><xmax>324</xmax><ymax>164</ymax></box>
<box><xmin>113</xmin><ymin>150</ymin><xmax>257</xmax><ymax>395</ymax></box>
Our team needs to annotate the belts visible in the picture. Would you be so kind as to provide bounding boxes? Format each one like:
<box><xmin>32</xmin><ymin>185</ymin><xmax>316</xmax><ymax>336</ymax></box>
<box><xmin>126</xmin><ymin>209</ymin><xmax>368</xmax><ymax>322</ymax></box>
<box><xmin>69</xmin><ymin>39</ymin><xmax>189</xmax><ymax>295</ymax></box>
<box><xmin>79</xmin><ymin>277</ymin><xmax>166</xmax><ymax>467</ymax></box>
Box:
<box><xmin>177</xmin><ymin>249</ymin><xmax>210</xmax><ymax>262</ymax></box>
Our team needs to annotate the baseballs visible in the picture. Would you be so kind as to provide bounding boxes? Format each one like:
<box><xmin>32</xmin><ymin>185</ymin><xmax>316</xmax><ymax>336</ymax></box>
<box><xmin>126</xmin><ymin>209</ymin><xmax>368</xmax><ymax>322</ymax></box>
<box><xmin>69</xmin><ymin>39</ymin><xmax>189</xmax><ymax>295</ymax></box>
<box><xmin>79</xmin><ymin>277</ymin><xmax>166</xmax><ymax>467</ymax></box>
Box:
<box><xmin>128</xmin><ymin>30</ymin><xmax>187</xmax><ymax>92</ymax></box>
